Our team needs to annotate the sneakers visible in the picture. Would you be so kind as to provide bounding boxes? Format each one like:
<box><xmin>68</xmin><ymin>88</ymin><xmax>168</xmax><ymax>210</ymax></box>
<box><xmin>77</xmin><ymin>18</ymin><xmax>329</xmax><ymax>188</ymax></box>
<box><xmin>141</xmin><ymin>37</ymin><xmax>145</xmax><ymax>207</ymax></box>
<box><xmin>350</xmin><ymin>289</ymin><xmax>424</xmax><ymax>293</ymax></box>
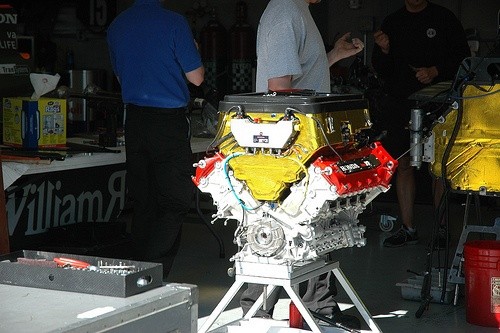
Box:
<box><xmin>432</xmin><ymin>232</ymin><xmax>450</xmax><ymax>250</ymax></box>
<box><xmin>383</xmin><ymin>224</ymin><xmax>419</xmax><ymax>247</ymax></box>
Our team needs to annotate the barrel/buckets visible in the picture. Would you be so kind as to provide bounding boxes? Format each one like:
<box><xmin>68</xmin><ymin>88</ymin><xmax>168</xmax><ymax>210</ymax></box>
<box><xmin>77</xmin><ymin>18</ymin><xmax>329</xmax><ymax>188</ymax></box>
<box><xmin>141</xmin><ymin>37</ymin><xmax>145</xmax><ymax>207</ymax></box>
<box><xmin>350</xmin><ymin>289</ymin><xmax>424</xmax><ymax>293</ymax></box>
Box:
<box><xmin>463</xmin><ymin>240</ymin><xmax>500</xmax><ymax>328</ymax></box>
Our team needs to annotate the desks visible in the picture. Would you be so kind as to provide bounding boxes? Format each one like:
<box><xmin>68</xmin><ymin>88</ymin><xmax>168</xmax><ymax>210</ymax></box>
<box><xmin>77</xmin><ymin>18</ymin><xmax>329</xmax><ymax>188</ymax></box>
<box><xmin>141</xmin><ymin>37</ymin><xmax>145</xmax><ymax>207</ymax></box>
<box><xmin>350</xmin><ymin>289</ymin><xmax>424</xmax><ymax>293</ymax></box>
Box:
<box><xmin>0</xmin><ymin>138</ymin><xmax>227</xmax><ymax>259</ymax></box>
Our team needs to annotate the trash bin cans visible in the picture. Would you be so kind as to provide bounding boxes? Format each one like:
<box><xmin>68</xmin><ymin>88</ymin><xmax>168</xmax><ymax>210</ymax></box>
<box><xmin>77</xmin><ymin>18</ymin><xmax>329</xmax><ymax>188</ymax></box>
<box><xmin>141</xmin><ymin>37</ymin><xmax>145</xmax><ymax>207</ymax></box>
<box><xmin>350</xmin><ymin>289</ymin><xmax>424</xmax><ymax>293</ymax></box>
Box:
<box><xmin>463</xmin><ymin>240</ymin><xmax>500</xmax><ymax>328</ymax></box>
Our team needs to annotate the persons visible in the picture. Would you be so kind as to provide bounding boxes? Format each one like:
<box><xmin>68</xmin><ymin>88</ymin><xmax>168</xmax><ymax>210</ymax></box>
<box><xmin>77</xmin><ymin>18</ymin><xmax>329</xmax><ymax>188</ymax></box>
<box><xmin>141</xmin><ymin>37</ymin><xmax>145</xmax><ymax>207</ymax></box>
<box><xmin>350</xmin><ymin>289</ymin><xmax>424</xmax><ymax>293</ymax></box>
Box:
<box><xmin>239</xmin><ymin>0</ymin><xmax>364</xmax><ymax>331</ymax></box>
<box><xmin>373</xmin><ymin>0</ymin><xmax>472</xmax><ymax>248</ymax></box>
<box><xmin>107</xmin><ymin>0</ymin><xmax>206</xmax><ymax>280</ymax></box>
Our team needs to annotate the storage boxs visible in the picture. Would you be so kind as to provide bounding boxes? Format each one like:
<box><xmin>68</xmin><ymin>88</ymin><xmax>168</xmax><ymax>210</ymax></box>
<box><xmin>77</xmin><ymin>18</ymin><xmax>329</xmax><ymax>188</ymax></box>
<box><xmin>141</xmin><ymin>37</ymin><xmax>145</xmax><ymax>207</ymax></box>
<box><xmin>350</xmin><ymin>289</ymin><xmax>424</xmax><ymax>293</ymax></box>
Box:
<box><xmin>3</xmin><ymin>97</ymin><xmax>67</xmax><ymax>144</ymax></box>
